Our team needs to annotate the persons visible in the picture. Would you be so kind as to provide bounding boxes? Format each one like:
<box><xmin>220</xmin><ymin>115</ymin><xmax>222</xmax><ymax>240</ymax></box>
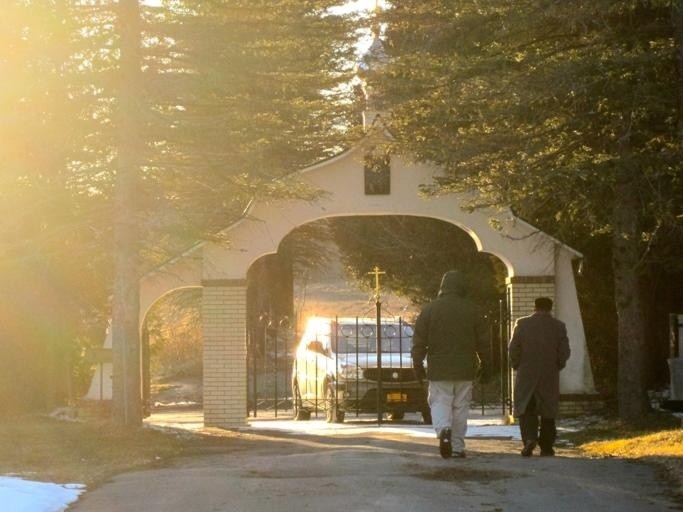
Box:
<box><xmin>410</xmin><ymin>270</ymin><xmax>492</xmax><ymax>460</ymax></box>
<box><xmin>505</xmin><ymin>296</ymin><xmax>572</xmax><ymax>458</ymax></box>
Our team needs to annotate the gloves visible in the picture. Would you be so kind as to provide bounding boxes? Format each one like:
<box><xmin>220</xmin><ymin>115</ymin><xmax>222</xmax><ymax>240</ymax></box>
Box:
<box><xmin>414</xmin><ymin>362</ymin><xmax>427</xmax><ymax>387</ymax></box>
<box><xmin>475</xmin><ymin>364</ymin><xmax>492</xmax><ymax>384</ymax></box>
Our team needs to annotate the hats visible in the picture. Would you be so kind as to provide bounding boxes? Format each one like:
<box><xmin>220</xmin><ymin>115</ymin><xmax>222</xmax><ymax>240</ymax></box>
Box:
<box><xmin>534</xmin><ymin>297</ymin><xmax>553</xmax><ymax>307</ymax></box>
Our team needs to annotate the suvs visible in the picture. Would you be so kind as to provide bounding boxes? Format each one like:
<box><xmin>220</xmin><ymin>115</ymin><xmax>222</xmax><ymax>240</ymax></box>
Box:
<box><xmin>288</xmin><ymin>316</ymin><xmax>432</xmax><ymax>426</ymax></box>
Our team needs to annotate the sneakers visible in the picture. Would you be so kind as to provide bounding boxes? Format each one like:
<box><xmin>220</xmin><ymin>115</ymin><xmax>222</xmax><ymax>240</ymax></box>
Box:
<box><xmin>539</xmin><ymin>448</ymin><xmax>555</xmax><ymax>458</ymax></box>
<box><xmin>521</xmin><ymin>439</ymin><xmax>537</xmax><ymax>457</ymax></box>
<box><xmin>451</xmin><ymin>450</ymin><xmax>466</xmax><ymax>459</ymax></box>
<box><xmin>438</xmin><ymin>428</ymin><xmax>452</xmax><ymax>459</ymax></box>
